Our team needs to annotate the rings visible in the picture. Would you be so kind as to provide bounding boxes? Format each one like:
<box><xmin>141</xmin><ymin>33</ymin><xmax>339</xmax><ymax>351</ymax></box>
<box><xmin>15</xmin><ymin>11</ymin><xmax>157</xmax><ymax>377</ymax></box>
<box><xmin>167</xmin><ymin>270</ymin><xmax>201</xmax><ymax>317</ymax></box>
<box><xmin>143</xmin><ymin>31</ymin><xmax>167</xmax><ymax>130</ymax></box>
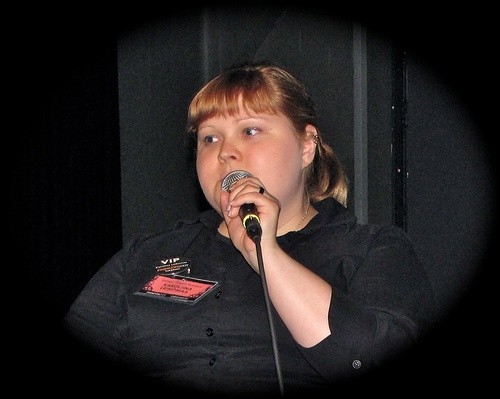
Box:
<box><xmin>259</xmin><ymin>185</ymin><xmax>266</xmax><ymax>195</ymax></box>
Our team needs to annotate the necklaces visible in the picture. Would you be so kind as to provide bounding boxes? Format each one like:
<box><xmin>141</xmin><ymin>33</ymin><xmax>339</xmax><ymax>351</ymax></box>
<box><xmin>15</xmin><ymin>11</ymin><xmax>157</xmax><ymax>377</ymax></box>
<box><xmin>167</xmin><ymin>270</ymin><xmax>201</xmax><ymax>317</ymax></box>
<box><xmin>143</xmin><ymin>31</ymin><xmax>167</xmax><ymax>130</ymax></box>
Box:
<box><xmin>295</xmin><ymin>189</ymin><xmax>314</xmax><ymax>229</ymax></box>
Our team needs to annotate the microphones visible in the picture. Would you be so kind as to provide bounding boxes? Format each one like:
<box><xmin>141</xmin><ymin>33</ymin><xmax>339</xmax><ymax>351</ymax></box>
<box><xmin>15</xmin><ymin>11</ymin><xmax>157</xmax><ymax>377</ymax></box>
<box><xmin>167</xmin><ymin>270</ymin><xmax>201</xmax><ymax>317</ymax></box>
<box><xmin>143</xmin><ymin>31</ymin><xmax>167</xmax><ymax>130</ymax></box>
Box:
<box><xmin>221</xmin><ymin>169</ymin><xmax>261</xmax><ymax>243</ymax></box>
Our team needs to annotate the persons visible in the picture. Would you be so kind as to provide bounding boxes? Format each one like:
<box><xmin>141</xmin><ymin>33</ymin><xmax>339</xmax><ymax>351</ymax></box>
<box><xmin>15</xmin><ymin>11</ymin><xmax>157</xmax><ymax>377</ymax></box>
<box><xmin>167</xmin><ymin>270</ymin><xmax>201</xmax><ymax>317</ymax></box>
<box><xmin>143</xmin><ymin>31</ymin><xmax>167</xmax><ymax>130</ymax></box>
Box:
<box><xmin>61</xmin><ymin>63</ymin><xmax>434</xmax><ymax>399</ymax></box>
